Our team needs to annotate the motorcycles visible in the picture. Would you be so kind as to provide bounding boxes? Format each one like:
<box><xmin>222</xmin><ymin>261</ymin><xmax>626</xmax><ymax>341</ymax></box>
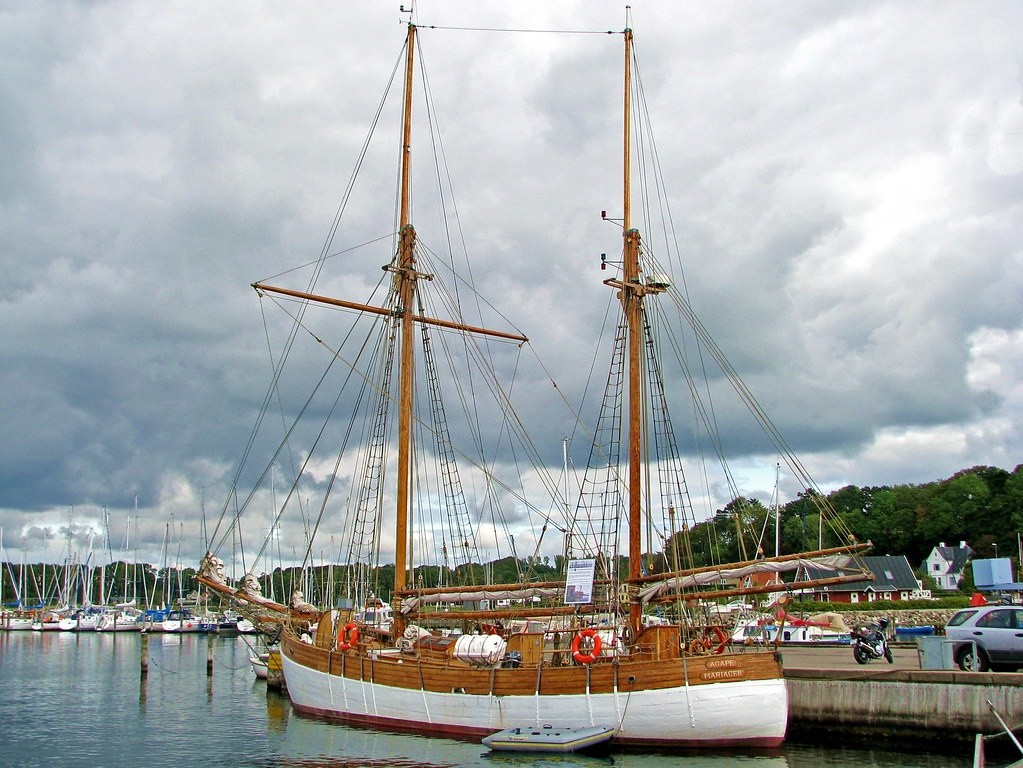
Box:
<box><xmin>849</xmin><ymin>618</ymin><xmax>894</xmax><ymax>665</ymax></box>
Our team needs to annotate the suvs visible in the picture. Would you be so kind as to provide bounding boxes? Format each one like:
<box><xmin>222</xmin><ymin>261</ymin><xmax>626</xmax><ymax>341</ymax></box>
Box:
<box><xmin>943</xmin><ymin>602</ymin><xmax>1023</xmax><ymax>673</ymax></box>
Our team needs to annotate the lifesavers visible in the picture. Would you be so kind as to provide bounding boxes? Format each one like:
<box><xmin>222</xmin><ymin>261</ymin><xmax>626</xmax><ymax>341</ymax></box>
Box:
<box><xmin>572</xmin><ymin>630</ymin><xmax>601</xmax><ymax>663</ymax></box>
<box><xmin>339</xmin><ymin>623</ymin><xmax>358</xmax><ymax>650</ymax></box>
<box><xmin>705</xmin><ymin>627</ymin><xmax>726</xmax><ymax>654</ymax></box>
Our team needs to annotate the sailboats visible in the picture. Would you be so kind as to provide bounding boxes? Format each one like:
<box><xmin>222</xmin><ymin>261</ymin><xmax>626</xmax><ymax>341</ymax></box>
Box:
<box><xmin>0</xmin><ymin>480</ymin><xmax>264</xmax><ymax>635</ymax></box>
<box><xmin>180</xmin><ymin>0</ymin><xmax>878</xmax><ymax>753</ymax></box>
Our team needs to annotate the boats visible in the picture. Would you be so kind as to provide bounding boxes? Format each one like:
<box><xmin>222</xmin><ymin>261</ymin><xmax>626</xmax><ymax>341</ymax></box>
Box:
<box><xmin>730</xmin><ymin>616</ymin><xmax>850</xmax><ymax>642</ymax></box>
<box><xmin>355</xmin><ymin>595</ymin><xmax>395</xmax><ymax>632</ymax></box>
